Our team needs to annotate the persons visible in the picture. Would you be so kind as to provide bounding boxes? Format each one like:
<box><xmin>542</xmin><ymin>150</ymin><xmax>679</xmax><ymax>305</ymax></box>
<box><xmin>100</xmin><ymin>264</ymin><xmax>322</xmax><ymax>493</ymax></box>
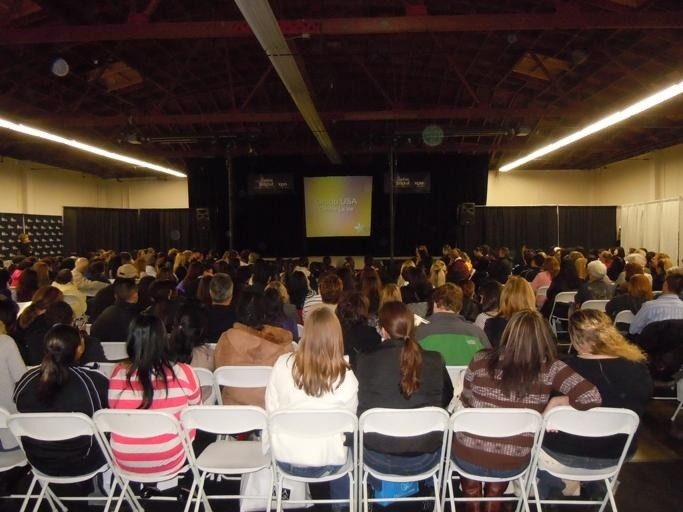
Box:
<box><xmin>546</xmin><ymin>251</ymin><xmax>584</xmax><ymax>299</ymax></box>
<box><xmin>201</xmin><ymin>249</ymin><xmax>220</xmax><ymax>263</ymax></box>
<box><xmin>378</xmin><ymin>283</ymin><xmax>401</xmax><ymax>313</ymax></box>
<box><xmin>398</xmin><ymin>259</ymin><xmax>415</xmax><ymax>288</ymax></box>
<box><xmin>528</xmin><ymin>256</ymin><xmax>561</xmax><ymax>308</ymax></box>
<box><xmin>134</xmin><ymin>247</ymin><xmax>164</xmax><ymax>263</ymax></box>
<box><xmin>458</xmin><ymin>281</ymin><xmax>484</xmax><ymax>320</ymax></box>
<box><xmin>606</xmin><ymin>274</ymin><xmax>654</xmax><ymax>331</ymax></box>
<box><xmin>349</xmin><ymin>269</ymin><xmax>382</xmax><ymax>313</ymax></box>
<box><xmin>339</xmin><ymin>269</ymin><xmax>355</xmax><ymax>289</ymax></box>
<box><xmin>651</xmin><ymin>253</ymin><xmax>671</xmax><ymax>268</ymax></box>
<box><xmin>450</xmin><ymin>310</ymin><xmax>601</xmax><ymax>512</ymax></box>
<box><xmin>428</xmin><ymin>259</ymin><xmax>447</xmax><ymax>289</ymax></box>
<box><xmin>412</xmin><ymin>283</ymin><xmax>493</xmax><ymax>366</ymax></box>
<box><xmin>216</xmin><ymin>291</ymin><xmax>294</xmax><ymax>406</ymax></box>
<box><xmin>1</xmin><ymin>270</ymin><xmax>20</xmax><ymax>313</ymax></box>
<box><xmin>294</xmin><ymin>256</ymin><xmax>310</xmax><ymax>275</ymax></box>
<box><xmin>91</xmin><ymin>264</ymin><xmax>138</xmax><ymax>319</ymax></box>
<box><xmin>538</xmin><ymin>308</ymin><xmax>652</xmax><ymax>501</ymax></box>
<box><xmin>652</xmin><ymin>258</ymin><xmax>673</xmax><ymax>290</ymax></box>
<box><xmin>228</xmin><ymin>258</ymin><xmax>247</xmax><ymax>283</ymax></box>
<box><xmin>145</xmin><ymin>278</ymin><xmax>181</xmax><ymax>331</ymax></box>
<box><xmin>313</xmin><ymin>258</ymin><xmax>384</xmax><ymax>269</ymax></box>
<box><xmin>190</xmin><ymin>248</ymin><xmax>200</xmax><ymax>263</ymax></box>
<box><xmin>167</xmin><ymin>305</ymin><xmax>215</xmax><ymax>370</ymax></box>
<box><xmin>173</xmin><ymin>253</ymin><xmax>186</xmax><ymax>280</ymax></box>
<box><xmin>51</xmin><ymin>269</ymin><xmax>87</xmax><ymax>317</ymax></box>
<box><xmin>17</xmin><ymin>285</ymin><xmax>64</xmax><ymax>330</ymax></box>
<box><xmin>486</xmin><ymin>243</ymin><xmax>496</xmax><ymax>270</ymax></box>
<box><xmin>1</xmin><ymin>257</ymin><xmax>32</xmax><ymax>269</ymax></box>
<box><xmin>117</xmin><ymin>252</ymin><xmax>137</xmax><ymax>278</ymax></box>
<box><xmin>334</xmin><ymin>293</ymin><xmax>382</xmax><ymax>370</ymax></box>
<box><xmin>134</xmin><ymin>258</ymin><xmax>157</xmax><ymax>284</ymax></box>
<box><xmin>598</xmin><ymin>251</ymin><xmax>623</xmax><ymax>281</ymax></box>
<box><xmin>358</xmin><ymin>301</ymin><xmax>454</xmax><ymax>510</ymax></box>
<box><xmin>183</xmin><ymin>264</ymin><xmax>217</xmax><ymax>304</ymax></box>
<box><xmin>255</xmin><ymin>288</ymin><xmax>299</xmax><ymax>341</ymax></box>
<box><xmin>584</xmin><ymin>245</ymin><xmax>614</xmax><ymax>255</ymax></box>
<box><xmin>613</xmin><ymin>262</ymin><xmax>644</xmax><ymax>296</ymax></box>
<box><xmin>0</xmin><ymin>299</ymin><xmax>28</xmax><ymax>453</ymax></box>
<box><xmin>513</xmin><ymin>250</ymin><xmax>532</xmax><ymax>277</ymax></box>
<box><xmin>250</xmin><ymin>255</ymin><xmax>293</xmax><ymax>274</ymax></box>
<box><xmin>18</xmin><ymin>269</ymin><xmax>39</xmax><ymax>300</ymax></box>
<box><xmin>614</xmin><ymin>246</ymin><xmax>626</xmax><ymax>264</ymax></box>
<box><xmin>88</xmin><ymin>247</ymin><xmax>120</xmax><ymax>259</ymax></box>
<box><xmin>90</xmin><ymin>279</ymin><xmax>144</xmax><ymax>343</ymax></box>
<box><xmin>475</xmin><ymin>279</ymin><xmax>503</xmax><ymax>329</ymax></box>
<box><xmin>493</xmin><ymin>248</ymin><xmax>512</xmax><ymax>277</ymax></box>
<box><xmin>472</xmin><ymin>246</ymin><xmax>488</xmax><ymax>278</ymax></box>
<box><xmin>212</xmin><ymin>249</ymin><xmax>238</xmax><ymax>272</ymax></box>
<box><xmin>485</xmin><ymin>276</ymin><xmax>536</xmax><ymax>346</ymax></box>
<box><xmin>32</xmin><ymin>262</ymin><xmax>50</xmax><ymax>284</ymax></box>
<box><xmin>629</xmin><ymin>266</ymin><xmax>683</xmax><ymax>334</ymax></box>
<box><xmin>150</xmin><ymin>264</ymin><xmax>175</xmax><ymax>277</ymax></box>
<box><xmin>197</xmin><ymin>274</ymin><xmax>213</xmax><ymax>302</ymax></box>
<box><xmin>109</xmin><ymin>312</ymin><xmax>201</xmax><ymax>503</ymax></box>
<box><xmin>70</xmin><ymin>257</ymin><xmax>110</xmax><ymax>288</ymax></box>
<box><xmin>454</xmin><ymin>248</ymin><xmax>472</xmax><ymax>275</ymax></box>
<box><xmin>200</xmin><ymin>273</ymin><xmax>236</xmax><ymax>344</ymax></box>
<box><xmin>440</xmin><ymin>241</ymin><xmax>456</xmax><ymax>263</ymax></box>
<box><xmin>264</xmin><ymin>307</ymin><xmax>359</xmax><ymax>511</ymax></box>
<box><xmin>46</xmin><ymin>254</ymin><xmax>75</xmax><ymax>268</ymax></box>
<box><xmin>447</xmin><ymin>248</ymin><xmax>470</xmax><ymax>283</ymax></box>
<box><xmin>87</xmin><ymin>260</ymin><xmax>112</xmax><ymax>283</ymax></box>
<box><xmin>627</xmin><ymin>247</ymin><xmax>654</xmax><ymax>265</ymax></box>
<box><xmin>303</xmin><ymin>274</ymin><xmax>343</xmax><ymax>323</ymax></box>
<box><xmin>12</xmin><ymin>324</ymin><xmax>109</xmax><ymax>475</ymax></box>
<box><xmin>264</xmin><ymin>280</ymin><xmax>300</xmax><ymax>323</ymax></box>
<box><xmin>165</xmin><ymin>248</ymin><xmax>180</xmax><ymax>275</ymax></box>
<box><xmin>411</xmin><ymin>240</ymin><xmax>433</xmax><ymax>281</ymax></box>
<box><xmin>406</xmin><ymin>280</ymin><xmax>433</xmax><ymax>317</ymax></box>
<box><xmin>519</xmin><ymin>245</ymin><xmax>586</xmax><ymax>255</ymax></box>
<box><xmin>576</xmin><ymin>260</ymin><xmax>616</xmax><ymax>309</ymax></box>
<box><xmin>183</xmin><ymin>250</ymin><xmax>192</xmax><ymax>268</ymax></box>
<box><xmin>30</xmin><ymin>301</ymin><xmax>109</xmax><ymax>363</ymax></box>
<box><xmin>287</xmin><ymin>271</ymin><xmax>317</xmax><ymax>308</ymax></box>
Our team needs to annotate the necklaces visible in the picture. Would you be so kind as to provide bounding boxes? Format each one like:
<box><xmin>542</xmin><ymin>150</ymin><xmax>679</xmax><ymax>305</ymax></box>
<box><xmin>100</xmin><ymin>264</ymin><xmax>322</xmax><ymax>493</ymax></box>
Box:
<box><xmin>581</xmin><ymin>352</ymin><xmax>591</xmax><ymax>354</ymax></box>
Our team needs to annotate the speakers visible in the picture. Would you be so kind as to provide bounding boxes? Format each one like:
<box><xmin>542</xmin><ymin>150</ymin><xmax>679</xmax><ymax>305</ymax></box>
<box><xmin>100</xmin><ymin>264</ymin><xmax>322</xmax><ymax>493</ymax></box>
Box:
<box><xmin>196</xmin><ymin>208</ymin><xmax>210</xmax><ymax>229</ymax></box>
<box><xmin>459</xmin><ymin>203</ymin><xmax>476</xmax><ymax>226</ymax></box>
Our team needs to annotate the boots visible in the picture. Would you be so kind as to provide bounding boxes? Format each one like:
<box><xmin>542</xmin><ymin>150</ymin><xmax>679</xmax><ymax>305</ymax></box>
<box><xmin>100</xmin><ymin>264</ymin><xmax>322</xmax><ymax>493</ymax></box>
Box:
<box><xmin>485</xmin><ymin>480</ymin><xmax>505</xmax><ymax>512</ymax></box>
<box><xmin>457</xmin><ymin>476</ymin><xmax>480</xmax><ymax>510</ymax></box>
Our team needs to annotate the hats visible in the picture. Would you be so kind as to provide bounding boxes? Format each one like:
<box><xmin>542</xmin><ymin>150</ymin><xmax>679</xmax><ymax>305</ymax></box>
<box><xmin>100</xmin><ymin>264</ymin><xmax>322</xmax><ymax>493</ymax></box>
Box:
<box><xmin>117</xmin><ymin>264</ymin><xmax>137</xmax><ymax>278</ymax></box>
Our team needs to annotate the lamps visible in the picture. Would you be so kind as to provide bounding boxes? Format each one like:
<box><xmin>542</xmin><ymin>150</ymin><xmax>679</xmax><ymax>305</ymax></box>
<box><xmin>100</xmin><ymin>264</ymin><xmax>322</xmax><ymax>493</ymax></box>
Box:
<box><xmin>51</xmin><ymin>57</ymin><xmax>76</xmax><ymax>78</ymax></box>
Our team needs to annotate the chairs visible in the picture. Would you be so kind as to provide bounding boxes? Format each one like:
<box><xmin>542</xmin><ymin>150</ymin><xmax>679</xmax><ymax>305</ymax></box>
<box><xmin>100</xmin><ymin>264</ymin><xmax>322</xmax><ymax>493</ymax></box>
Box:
<box><xmin>613</xmin><ymin>310</ymin><xmax>635</xmax><ymax>334</ymax></box>
<box><xmin>213</xmin><ymin>366</ymin><xmax>273</xmax><ymax>482</ymax></box>
<box><xmin>268</xmin><ymin>410</ymin><xmax>358</xmax><ymax>512</ymax></box>
<box><xmin>0</xmin><ymin>408</ymin><xmax>58</xmax><ymax>512</ymax></box>
<box><xmin>549</xmin><ymin>291</ymin><xmax>577</xmax><ymax>339</ymax></box>
<box><xmin>191</xmin><ymin>366</ymin><xmax>222</xmax><ymax>480</ymax></box>
<box><xmin>101</xmin><ymin>342</ymin><xmax>130</xmax><ymax>361</ymax></box>
<box><xmin>441</xmin><ymin>408</ymin><xmax>542</xmax><ymax>512</ymax></box>
<box><xmin>84</xmin><ymin>362</ymin><xmax>119</xmax><ymax>380</ymax></box>
<box><xmin>568</xmin><ymin>300</ymin><xmax>610</xmax><ymax>355</ymax></box>
<box><xmin>205</xmin><ymin>342</ymin><xmax>217</xmax><ymax>349</ymax></box>
<box><xmin>359</xmin><ymin>406</ymin><xmax>450</xmax><ymax>512</ymax></box>
<box><xmin>180</xmin><ymin>406</ymin><xmax>282</xmax><ymax>512</ymax></box>
<box><xmin>521</xmin><ymin>406</ymin><xmax>640</xmax><ymax>512</ymax></box>
<box><xmin>7</xmin><ymin>413</ymin><xmax>138</xmax><ymax>512</ymax></box>
<box><xmin>445</xmin><ymin>365</ymin><xmax>469</xmax><ymax>413</ymax></box>
<box><xmin>468</xmin><ymin>269</ymin><xmax>476</xmax><ymax>279</ymax></box>
<box><xmin>92</xmin><ymin>409</ymin><xmax>209</xmax><ymax>512</ymax></box>
<box><xmin>652</xmin><ymin>291</ymin><xmax>663</xmax><ymax>296</ymax></box>
<box><xmin>652</xmin><ymin>379</ymin><xmax>683</xmax><ymax>422</ymax></box>
